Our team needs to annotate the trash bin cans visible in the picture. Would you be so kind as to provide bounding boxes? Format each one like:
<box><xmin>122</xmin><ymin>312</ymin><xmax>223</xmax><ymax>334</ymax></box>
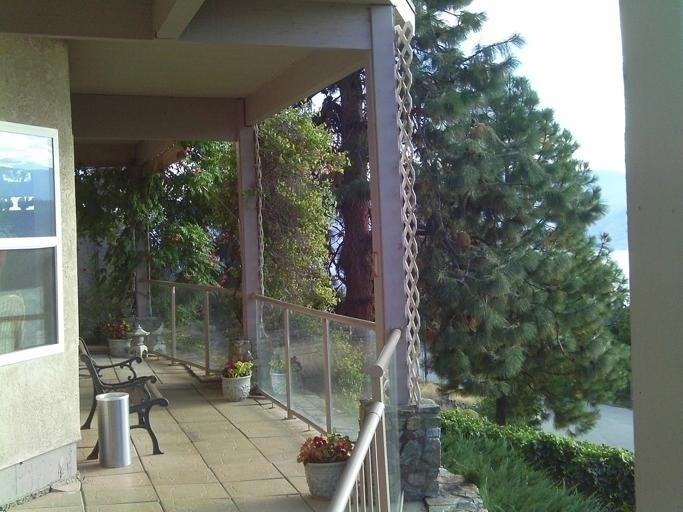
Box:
<box><xmin>95</xmin><ymin>392</ymin><xmax>132</xmax><ymax>468</ymax></box>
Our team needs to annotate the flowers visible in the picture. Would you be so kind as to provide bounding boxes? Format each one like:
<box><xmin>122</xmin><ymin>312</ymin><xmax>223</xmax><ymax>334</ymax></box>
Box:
<box><xmin>222</xmin><ymin>359</ymin><xmax>254</xmax><ymax>378</ymax></box>
<box><xmin>267</xmin><ymin>356</ymin><xmax>303</xmax><ymax>373</ymax></box>
<box><xmin>296</xmin><ymin>427</ymin><xmax>353</xmax><ymax>466</ymax></box>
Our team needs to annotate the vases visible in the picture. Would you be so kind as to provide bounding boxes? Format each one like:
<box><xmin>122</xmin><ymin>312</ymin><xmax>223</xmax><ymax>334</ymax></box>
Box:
<box><xmin>270</xmin><ymin>371</ymin><xmax>298</xmax><ymax>396</ymax></box>
<box><xmin>220</xmin><ymin>370</ymin><xmax>253</xmax><ymax>402</ymax></box>
<box><xmin>303</xmin><ymin>451</ymin><xmax>352</xmax><ymax>501</ymax></box>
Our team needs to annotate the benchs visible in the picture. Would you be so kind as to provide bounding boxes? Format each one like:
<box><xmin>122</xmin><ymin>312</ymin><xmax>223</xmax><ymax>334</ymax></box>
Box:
<box><xmin>79</xmin><ymin>336</ymin><xmax>169</xmax><ymax>460</ymax></box>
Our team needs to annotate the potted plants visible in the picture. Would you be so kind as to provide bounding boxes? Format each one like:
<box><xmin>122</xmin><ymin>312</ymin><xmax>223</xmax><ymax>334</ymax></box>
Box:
<box><xmin>104</xmin><ymin>319</ymin><xmax>133</xmax><ymax>357</ymax></box>
